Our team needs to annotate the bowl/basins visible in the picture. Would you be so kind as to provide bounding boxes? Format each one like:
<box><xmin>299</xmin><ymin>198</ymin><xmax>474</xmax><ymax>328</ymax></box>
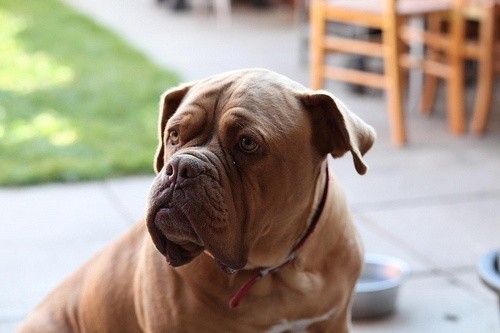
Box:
<box><xmin>352</xmin><ymin>258</ymin><xmax>403</xmax><ymax>317</ymax></box>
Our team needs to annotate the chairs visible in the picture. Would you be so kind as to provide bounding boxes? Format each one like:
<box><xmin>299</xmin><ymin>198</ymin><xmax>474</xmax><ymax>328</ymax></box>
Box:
<box><xmin>309</xmin><ymin>0</ymin><xmax>500</xmax><ymax>146</ymax></box>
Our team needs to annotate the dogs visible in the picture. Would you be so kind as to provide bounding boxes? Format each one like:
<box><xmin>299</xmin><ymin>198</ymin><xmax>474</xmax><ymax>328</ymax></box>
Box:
<box><xmin>9</xmin><ymin>67</ymin><xmax>376</xmax><ymax>333</ymax></box>
